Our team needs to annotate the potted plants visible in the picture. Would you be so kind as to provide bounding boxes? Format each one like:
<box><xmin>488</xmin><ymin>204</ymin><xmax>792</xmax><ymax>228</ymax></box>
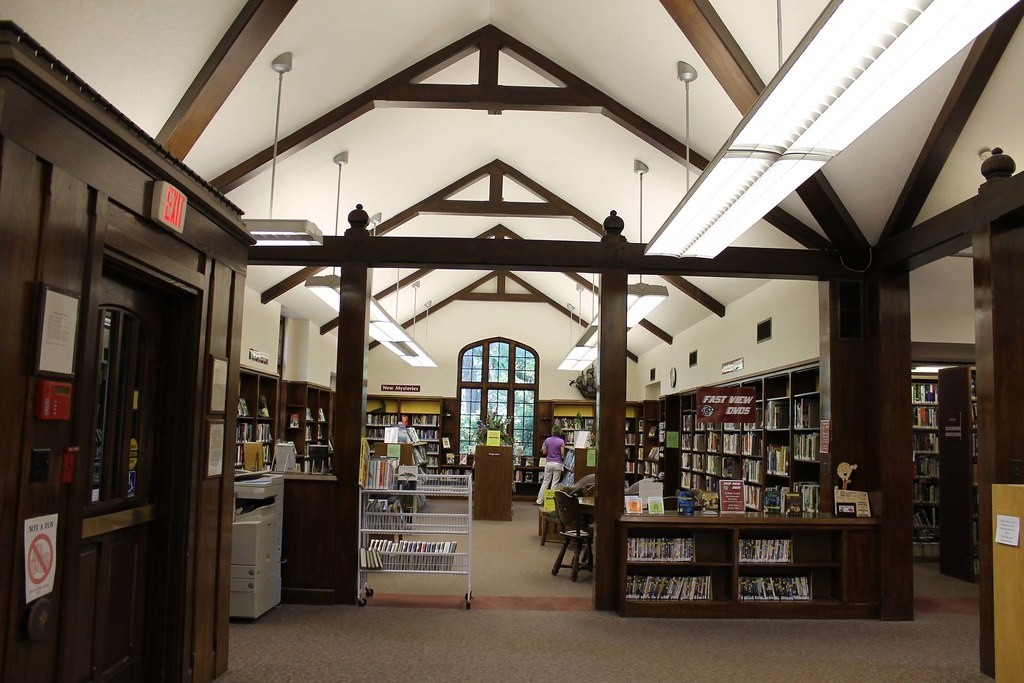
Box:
<box><xmin>568</xmin><ymin>364</ymin><xmax>597</xmax><ymax>399</ymax></box>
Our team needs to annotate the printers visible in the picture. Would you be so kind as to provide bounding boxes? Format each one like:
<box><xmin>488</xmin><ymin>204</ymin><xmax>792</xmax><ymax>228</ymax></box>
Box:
<box><xmin>233</xmin><ymin>469</ymin><xmax>284</xmax><ymax>617</ymax></box>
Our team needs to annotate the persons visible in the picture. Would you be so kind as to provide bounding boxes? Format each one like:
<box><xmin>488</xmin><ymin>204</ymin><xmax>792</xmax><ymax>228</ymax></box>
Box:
<box><xmin>536</xmin><ymin>425</ymin><xmax>564</xmax><ymax>505</ymax></box>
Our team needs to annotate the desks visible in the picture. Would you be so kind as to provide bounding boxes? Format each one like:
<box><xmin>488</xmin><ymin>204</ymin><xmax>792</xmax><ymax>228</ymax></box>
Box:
<box><xmin>577</xmin><ymin>496</ymin><xmax>648</xmax><ymax>563</ymax></box>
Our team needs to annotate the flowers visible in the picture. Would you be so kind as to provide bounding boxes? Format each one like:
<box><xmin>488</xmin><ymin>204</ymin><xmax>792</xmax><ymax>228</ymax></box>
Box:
<box><xmin>475</xmin><ymin>412</ymin><xmax>514</xmax><ymax>446</ymax></box>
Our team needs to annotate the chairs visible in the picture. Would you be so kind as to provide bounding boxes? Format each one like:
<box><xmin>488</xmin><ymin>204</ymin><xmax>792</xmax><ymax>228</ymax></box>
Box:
<box><xmin>539</xmin><ymin>486</ymin><xmax>584</xmax><ymax>547</ymax></box>
<box><xmin>551</xmin><ymin>491</ymin><xmax>593</xmax><ymax>582</ymax></box>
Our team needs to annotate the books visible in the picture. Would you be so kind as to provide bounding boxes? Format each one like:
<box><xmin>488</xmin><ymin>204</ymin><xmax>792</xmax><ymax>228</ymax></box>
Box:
<box><xmin>235</xmin><ymin>397</ymin><xmax>323</xmax><ymax>473</ymax></box>
<box><xmin>361</xmin><ymin>539</ymin><xmax>458</xmax><ymax>571</ymax></box>
<box><xmin>738</xmin><ymin>576</ymin><xmax>810</xmax><ymax>600</ymax></box>
<box><xmin>625</xmin><ymin>398</ymin><xmax>821</xmax><ymax>515</ymax></box>
<box><xmin>555</xmin><ymin>417</ymin><xmax>593</xmax><ymax>487</ymax></box>
<box><xmin>627</xmin><ymin>537</ymin><xmax>692</xmax><ymax>561</ymax></box>
<box><xmin>359</xmin><ymin>414</ymin><xmax>454</xmax><ymax>489</ymax></box>
<box><xmin>366</xmin><ymin>495</ymin><xmax>426</xmax><ymax>530</ymax></box>
<box><xmin>739</xmin><ymin>539</ymin><xmax>791</xmax><ymax>562</ymax></box>
<box><xmin>911</xmin><ymin>376</ymin><xmax>980</xmax><ymax>575</ymax></box>
<box><xmin>626</xmin><ymin>576</ymin><xmax>710</xmax><ymax>599</ymax></box>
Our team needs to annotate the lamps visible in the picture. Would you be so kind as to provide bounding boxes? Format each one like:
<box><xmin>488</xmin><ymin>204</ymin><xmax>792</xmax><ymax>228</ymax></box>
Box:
<box><xmin>304</xmin><ymin>151</ymin><xmax>441</xmax><ymax>369</ymax></box>
<box><xmin>643</xmin><ymin>0</ymin><xmax>1023</xmax><ymax>260</ymax></box>
<box><xmin>556</xmin><ymin>159</ymin><xmax>669</xmax><ymax>372</ymax></box>
<box><xmin>242</xmin><ymin>51</ymin><xmax>324</xmax><ymax>247</ymax></box>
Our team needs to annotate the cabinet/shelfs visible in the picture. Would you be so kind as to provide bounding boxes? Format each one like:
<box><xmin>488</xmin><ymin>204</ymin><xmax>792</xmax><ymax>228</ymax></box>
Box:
<box><xmin>534</xmin><ymin>357</ymin><xmax>978</xmax><ymax>622</ymax></box>
<box><xmin>234</xmin><ymin>364</ymin><xmax>562</xmax><ymax>502</ymax></box>
<box><xmin>356</xmin><ymin>474</ymin><xmax>474</xmax><ymax>610</ymax></box>
